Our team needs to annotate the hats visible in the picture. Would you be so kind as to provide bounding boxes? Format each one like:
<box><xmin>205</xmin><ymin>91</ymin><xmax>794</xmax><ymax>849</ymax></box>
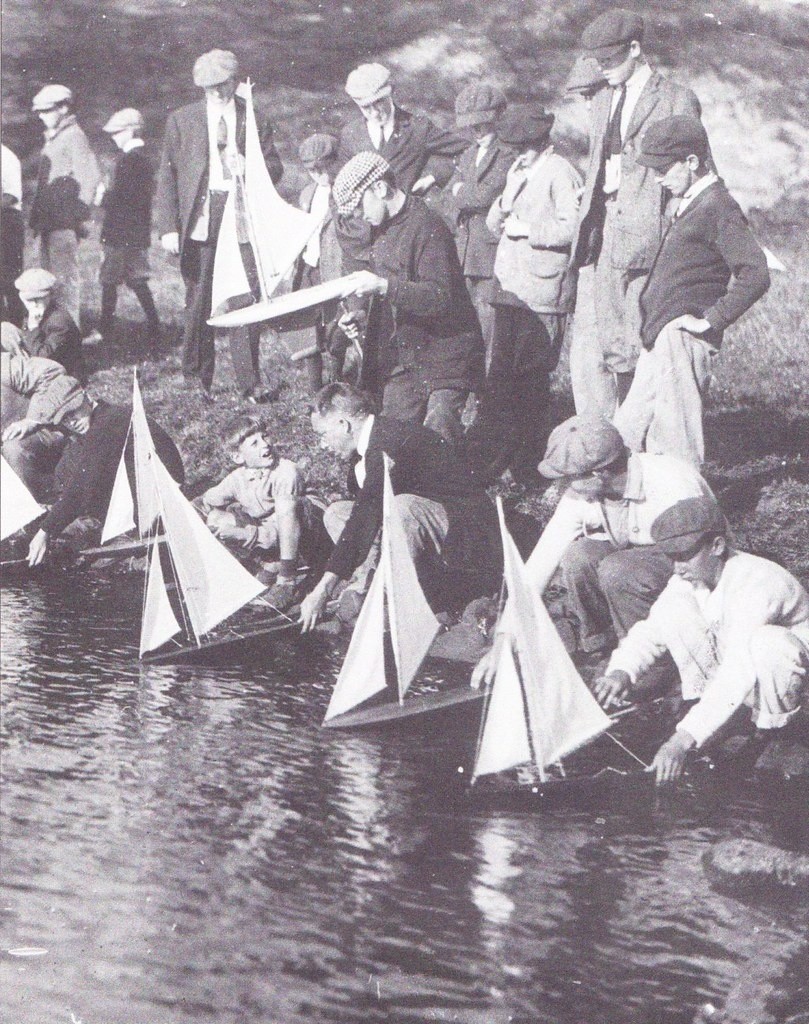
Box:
<box><xmin>565</xmin><ymin>57</ymin><xmax>608</xmax><ymax>93</ymax></box>
<box><xmin>40</xmin><ymin>375</ymin><xmax>83</xmax><ymax>422</ymax></box>
<box><xmin>193</xmin><ymin>49</ymin><xmax>239</xmax><ymax>87</ymax></box>
<box><xmin>455</xmin><ymin>85</ymin><xmax>507</xmax><ymax>129</ymax></box>
<box><xmin>634</xmin><ymin>113</ymin><xmax>709</xmax><ymax>169</ymax></box>
<box><xmin>537</xmin><ymin>414</ymin><xmax>623</xmax><ymax>481</ymax></box>
<box><xmin>580</xmin><ymin>9</ymin><xmax>644</xmax><ymax>63</ymax></box>
<box><xmin>300</xmin><ymin>134</ymin><xmax>337</xmax><ymax>169</ymax></box>
<box><xmin>31</xmin><ymin>84</ymin><xmax>71</xmax><ymax>112</ymax></box>
<box><xmin>102</xmin><ymin>108</ymin><xmax>143</xmax><ymax>133</ymax></box>
<box><xmin>14</xmin><ymin>267</ymin><xmax>56</xmax><ymax>298</ymax></box>
<box><xmin>496</xmin><ymin>102</ymin><xmax>556</xmax><ymax>147</ymax></box>
<box><xmin>344</xmin><ymin>63</ymin><xmax>392</xmax><ymax>108</ymax></box>
<box><xmin>332</xmin><ymin>152</ymin><xmax>391</xmax><ymax>214</ymax></box>
<box><xmin>651</xmin><ymin>497</ymin><xmax>726</xmax><ymax>553</ymax></box>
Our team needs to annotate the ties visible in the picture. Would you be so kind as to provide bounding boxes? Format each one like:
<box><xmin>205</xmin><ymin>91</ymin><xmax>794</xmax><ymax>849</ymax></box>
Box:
<box><xmin>379</xmin><ymin>126</ymin><xmax>386</xmax><ymax>153</ymax></box>
<box><xmin>606</xmin><ymin>83</ymin><xmax>626</xmax><ymax>154</ymax></box>
<box><xmin>216</xmin><ymin>116</ymin><xmax>227</xmax><ymax>152</ymax></box>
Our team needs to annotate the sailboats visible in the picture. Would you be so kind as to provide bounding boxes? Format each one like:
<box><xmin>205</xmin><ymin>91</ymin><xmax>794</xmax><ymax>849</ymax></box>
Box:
<box><xmin>1</xmin><ymin>455</ymin><xmax>65</xmax><ymax>572</ymax></box>
<box><xmin>128</xmin><ymin>445</ymin><xmax>319</xmax><ymax>666</ymax></box>
<box><xmin>76</xmin><ymin>365</ymin><xmax>220</xmax><ymax>561</ymax></box>
<box><xmin>323</xmin><ymin>449</ymin><xmax>496</xmax><ymax>730</ymax></box>
<box><xmin>455</xmin><ymin>488</ymin><xmax>695</xmax><ymax>800</ymax></box>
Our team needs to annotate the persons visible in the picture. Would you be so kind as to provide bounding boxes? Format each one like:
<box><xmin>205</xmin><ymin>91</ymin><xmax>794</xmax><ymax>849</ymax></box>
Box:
<box><xmin>203</xmin><ymin>413</ymin><xmax>326</xmax><ymax>607</ymax></box>
<box><xmin>0</xmin><ymin>352</ymin><xmax>69</xmax><ymax>538</ymax></box>
<box><xmin>28</xmin><ymin>84</ymin><xmax>100</xmax><ymax>335</ymax></box>
<box><xmin>561</xmin><ymin>8</ymin><xmax>703</xmax><ymax>415</ymax></box>
<box><xmin>24</xmin><ymin>375</ymin><xmax>186</xmax><ymax>568</ymax></box>
<box><xmin>298</xmin><ymin>382</ymin><xmax>502</xmax><ymax>635</ymax></box>
<box><xmin>0</xmin><ymin>143</ymin><xmax>24</xmax><ymax>327</ymax></box>
<box><xmin>156</xmin><ymin>49</ymin><xmax>284</xmax><ymax>404</ymax></box>
<box><xmin>594</xmin><ymin>497</ymin><xmax>809</xmax><ymax>786</ymax></box>
<box><xmin>297</xmin><ymin>132</ymin><xmax>351</xmax><ymax>399</ymax></box>
<box><xmin>614</xmin><ymin>114</ymin><xmax>771</xmax><ymax>472</ymax></box>
<box><xmin>486</xmin><ymin>102</ymin><xmax>584</xmax><ymax>488</ymax></box>
<box><xmin>471</xmin><ymin>413</ymin><xmax>733</xmax><ymax>691</ymax></box>
<box><xmin>432</xmin><ymin>82</ymin><xmax>508</xmax><ymax>374</ymax></box>
<box><xmin>329</xmin><ymin>62</ymin><xmax>474</xmax><ymax>206</ymax></box>
<box><xmin>0</xmin><ymin>268</ymin><xmax>88</xmax><ymax>386</ymax></box>
<box><xmin>81</xmin><ymin>106</ymin><xmax>162</xmax><ymax>345</ymax></box>
<box><xmin>331</xmin><ymin>151</ymin><xmax>486</xmax><ymax>452</ymax></box>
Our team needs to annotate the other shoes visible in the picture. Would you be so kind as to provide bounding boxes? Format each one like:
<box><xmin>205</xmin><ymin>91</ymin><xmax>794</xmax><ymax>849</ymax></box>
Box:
<box><xmin>247</xmin><ymin>381</ymin><xmax>271</xmax><ymax>400</ymax></box>
<box><xmin>256</xmin><ymin>573</ymin><xmax>277</xmax><ymax>589</ymax></box>
<box><xmin>251</xmin><ymin>583</ymin><xmax>303</xmax><ymax>613</ymax></box>
<box><xmin>90</xmin><ymin>535</ymin><xmax>135</xmax><ymax>569</ymax></box>
